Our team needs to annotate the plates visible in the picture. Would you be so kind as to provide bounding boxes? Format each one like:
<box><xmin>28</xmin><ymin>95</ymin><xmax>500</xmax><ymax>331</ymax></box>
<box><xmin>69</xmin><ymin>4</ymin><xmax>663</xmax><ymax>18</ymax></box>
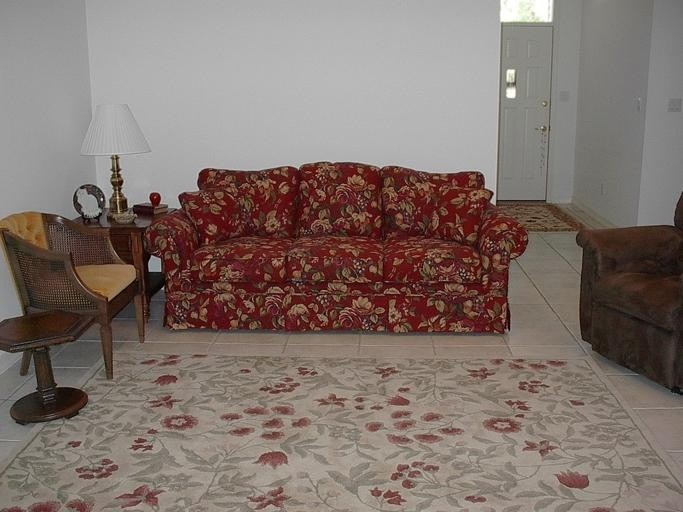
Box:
<box><xmin>74</xmin><ymin>184</ymin><xmax>106</xmax><ymax>217</ymax></box>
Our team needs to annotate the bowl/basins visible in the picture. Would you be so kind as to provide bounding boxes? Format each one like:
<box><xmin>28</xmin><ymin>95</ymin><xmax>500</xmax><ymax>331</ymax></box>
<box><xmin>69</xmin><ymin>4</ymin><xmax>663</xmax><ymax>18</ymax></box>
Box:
<box><xmin>113</xmin><ymin>214</ymin><xmax>137</xmax><ymax>224</ymax></box>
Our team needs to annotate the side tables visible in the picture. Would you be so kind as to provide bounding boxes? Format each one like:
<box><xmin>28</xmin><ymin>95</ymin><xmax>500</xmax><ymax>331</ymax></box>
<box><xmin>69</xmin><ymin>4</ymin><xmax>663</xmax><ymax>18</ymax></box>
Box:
<box><xmin>0</xmin><ymin>309</ymin><xmax>95</xmax><ymax>425</ymax></box>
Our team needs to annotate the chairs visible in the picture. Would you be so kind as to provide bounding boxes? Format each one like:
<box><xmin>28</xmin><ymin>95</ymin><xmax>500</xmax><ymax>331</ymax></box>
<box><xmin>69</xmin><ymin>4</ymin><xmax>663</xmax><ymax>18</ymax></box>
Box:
<box><xmin>0</xmin><ymin>211</ymin><xmax>145</xmax><ymax>380</ymax></box>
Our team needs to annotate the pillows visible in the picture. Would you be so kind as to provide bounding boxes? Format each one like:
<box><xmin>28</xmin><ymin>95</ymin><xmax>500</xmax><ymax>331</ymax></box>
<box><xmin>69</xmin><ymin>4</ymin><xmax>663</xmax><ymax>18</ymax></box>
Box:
<box><xmin>425</xmin><ymin>184</ymin><xmax>493</xmax><ymax>247</ymax></box>
<box><xmin>179</xmin><ymin>185</ymin><xmax>246</xmax><ymax>245</ymax></box>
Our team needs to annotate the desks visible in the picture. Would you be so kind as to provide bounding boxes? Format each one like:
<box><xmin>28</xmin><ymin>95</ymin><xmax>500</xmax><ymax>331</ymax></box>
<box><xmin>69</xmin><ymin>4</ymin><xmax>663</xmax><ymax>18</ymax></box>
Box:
<box><xmin>71</xmin><ymin>207</ymin><xmax>178</xmax><ymax>323</ymax></box>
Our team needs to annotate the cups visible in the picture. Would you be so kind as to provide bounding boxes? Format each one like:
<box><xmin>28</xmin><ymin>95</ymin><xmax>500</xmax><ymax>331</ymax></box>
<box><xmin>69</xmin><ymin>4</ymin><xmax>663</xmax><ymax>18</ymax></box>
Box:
<box><xmin>144</xmin><ymin>192</ymin><xmax>151</xmax><ymax>204</ymax></box>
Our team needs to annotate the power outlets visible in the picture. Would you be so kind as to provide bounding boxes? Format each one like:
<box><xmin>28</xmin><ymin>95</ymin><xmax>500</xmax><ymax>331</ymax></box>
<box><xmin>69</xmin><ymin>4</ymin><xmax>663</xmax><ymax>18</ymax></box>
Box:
<box><xmin>663</xmin><ymin>97</ymin><xmax>681</xmax><ymax>113</ymax></box>
<box><xmin>636</xmin><ymin>97</ymin><xmax>641</xmax><ymax>112</ymax></box>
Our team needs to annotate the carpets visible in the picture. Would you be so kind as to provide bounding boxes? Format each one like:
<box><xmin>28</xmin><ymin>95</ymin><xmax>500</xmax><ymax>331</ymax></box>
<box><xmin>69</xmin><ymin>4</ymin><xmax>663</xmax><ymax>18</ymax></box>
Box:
<box><xmin>0</xmin><ymin>354</ymin><xmax>683</xmax><ymax>512</ymax></box>
<box><xmin>496</xmin><ymin>204</ymin><xmax>587</xmax><ymax>232</ymax></box>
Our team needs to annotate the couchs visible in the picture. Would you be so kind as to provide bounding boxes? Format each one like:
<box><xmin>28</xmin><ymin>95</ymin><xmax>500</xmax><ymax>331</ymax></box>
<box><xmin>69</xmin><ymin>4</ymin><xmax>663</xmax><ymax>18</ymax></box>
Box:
<box><xmin>143</xmin><ymin>160</ymin><xmax>530</xmax><ymax>335</ymax></box>
<box><xmin>575</xmin><ymin>192</ymin><xmax>683</xmax><ymax>397</ymax></box>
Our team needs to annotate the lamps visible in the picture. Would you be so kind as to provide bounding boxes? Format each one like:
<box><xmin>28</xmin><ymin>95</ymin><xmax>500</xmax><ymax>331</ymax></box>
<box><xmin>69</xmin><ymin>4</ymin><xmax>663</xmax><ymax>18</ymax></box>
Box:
<box><xmin>79</xmin><ymin>104</ymin><xmax>152</xmax><ymax>214</ymax></box>
